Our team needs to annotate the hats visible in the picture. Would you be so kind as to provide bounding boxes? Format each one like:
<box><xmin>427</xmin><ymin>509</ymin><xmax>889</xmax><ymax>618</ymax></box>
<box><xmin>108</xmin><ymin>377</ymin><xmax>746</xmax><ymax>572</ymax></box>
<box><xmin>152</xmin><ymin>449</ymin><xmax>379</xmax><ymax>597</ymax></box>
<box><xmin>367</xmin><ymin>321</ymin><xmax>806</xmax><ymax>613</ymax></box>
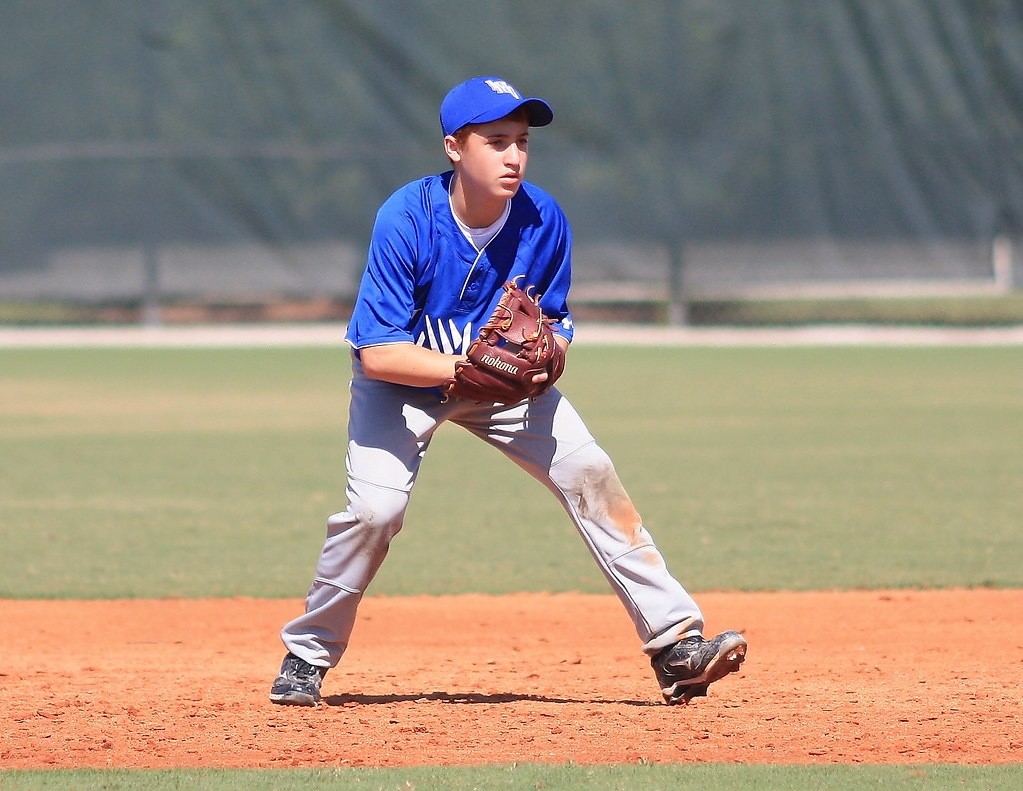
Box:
<box><xmin>440</xmin><ymin>78</ymin><xmax>553</xmax><ymax>139</ymax></box>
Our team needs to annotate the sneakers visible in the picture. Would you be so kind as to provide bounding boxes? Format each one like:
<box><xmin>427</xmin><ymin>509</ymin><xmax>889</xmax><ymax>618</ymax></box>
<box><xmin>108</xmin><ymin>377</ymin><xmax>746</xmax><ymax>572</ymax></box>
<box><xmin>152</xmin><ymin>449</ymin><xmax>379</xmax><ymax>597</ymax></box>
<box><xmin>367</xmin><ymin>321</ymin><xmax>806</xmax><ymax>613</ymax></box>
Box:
<box><xmin>653</xmin><ymin>631</ymin><xmax>748</xmax><ymax>705</ymax></box>
<box><xmin>269</xmin><ymin>653</ymin><xmax>329</xmax><ymax>706</ymax></box>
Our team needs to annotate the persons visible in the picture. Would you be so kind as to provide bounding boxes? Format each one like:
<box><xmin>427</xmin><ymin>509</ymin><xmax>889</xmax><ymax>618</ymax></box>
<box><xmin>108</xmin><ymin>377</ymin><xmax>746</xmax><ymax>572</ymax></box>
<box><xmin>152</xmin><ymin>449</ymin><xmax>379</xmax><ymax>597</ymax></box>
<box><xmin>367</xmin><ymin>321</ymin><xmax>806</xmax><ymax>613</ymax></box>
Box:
<box><xmin>270</xmin><ymin>78</ymin><xmax>746</xmax><ymax>706</ymax></box>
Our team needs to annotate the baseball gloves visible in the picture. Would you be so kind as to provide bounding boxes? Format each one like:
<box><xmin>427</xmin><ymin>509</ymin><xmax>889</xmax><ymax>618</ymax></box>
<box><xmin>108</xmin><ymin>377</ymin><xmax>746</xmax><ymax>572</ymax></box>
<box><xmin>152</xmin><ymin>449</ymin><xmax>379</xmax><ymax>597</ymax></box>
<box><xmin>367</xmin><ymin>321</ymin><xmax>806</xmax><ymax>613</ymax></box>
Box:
<box><xmin>441</xmin><ymin>282</ymin><xmax>565</xmax><ymax>405</ymax></box>
